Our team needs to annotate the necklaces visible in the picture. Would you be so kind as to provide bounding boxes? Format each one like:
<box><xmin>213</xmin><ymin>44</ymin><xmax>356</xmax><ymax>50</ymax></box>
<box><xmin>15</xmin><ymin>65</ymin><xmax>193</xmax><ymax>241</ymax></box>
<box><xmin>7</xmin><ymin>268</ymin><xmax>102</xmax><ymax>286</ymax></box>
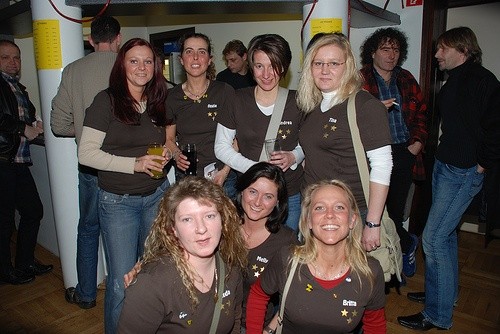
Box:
<box><xmin>186</xmin><ymin>82</ymin><xmax>208</xmax><ymax>98</ymax></box>
<box><xmin>316</xmin><ymin>269</ymin><xmax>340</xmax><ymax>279</ymax></box>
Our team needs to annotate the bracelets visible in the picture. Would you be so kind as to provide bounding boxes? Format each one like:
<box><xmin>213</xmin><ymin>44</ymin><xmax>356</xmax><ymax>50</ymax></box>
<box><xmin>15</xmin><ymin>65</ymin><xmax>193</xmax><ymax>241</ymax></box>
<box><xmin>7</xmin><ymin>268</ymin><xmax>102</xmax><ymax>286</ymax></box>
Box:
<box><xmin>223</xmin><ymin>168</ymin><xmax>227</xmax><ymax>175</ymax></box>
<box><xmin>265</xmin><ymin>327</ymin><xmax>275</xmax><ymax>334</ymax></box>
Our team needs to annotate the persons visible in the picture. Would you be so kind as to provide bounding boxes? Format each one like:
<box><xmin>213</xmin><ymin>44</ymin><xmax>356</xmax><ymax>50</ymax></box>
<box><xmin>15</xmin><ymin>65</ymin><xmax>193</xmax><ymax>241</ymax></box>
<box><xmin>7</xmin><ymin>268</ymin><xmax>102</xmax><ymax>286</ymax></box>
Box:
<box><xmin>217</xmin><ymin>39</ymin><xmax>258</xmax><ymax>89</ymax></box>
<box><xmin>296</xmin><ymin>32</ymin><xmax>393</xmax><ymax>253</ymax></box>
<box><xmin>165</xmin><ymin>33</ymin><xmax>234</xmax><ymax>182</ymax></box>
<box><xmin>214</xmin><ymin>34</ymin><xmax>306</xmax><ymax>232</ymax></box>
<box><xmin>117</xmin><ymin>176</ymin><xmax>246</xmax><ymax>334</ymax></box>
<box><xmin>124</xmin><ymin>162</ymin><xmax>300</xmax><ymax>334</ymax></box>
<box><xmin>359</xmin><ymin>28</ymin><xmax>429</xmax><ymax>276</ymax></box>
<box><xmin>51</xmin><ymin>15</ymin><xmax>122</xmax><ymax>309</ymax></box>
<box><xmin>397</xmin><ymin>27</ymin><xmax>500</xmax><ymax>329</ymax></box>
<box><xmin>78</xmin><ymin>38</ymin><xmax>170</xmax><ymax>334</ymax></box>
<box><xmin>0</xmin><ymin>40</ymin><xmax>54</xmax><ymax>284</ymax></box>
<box><xmin>247</xmin><ymin>180</ymin><xmax>387</xmax><ymax>334</ymax></box>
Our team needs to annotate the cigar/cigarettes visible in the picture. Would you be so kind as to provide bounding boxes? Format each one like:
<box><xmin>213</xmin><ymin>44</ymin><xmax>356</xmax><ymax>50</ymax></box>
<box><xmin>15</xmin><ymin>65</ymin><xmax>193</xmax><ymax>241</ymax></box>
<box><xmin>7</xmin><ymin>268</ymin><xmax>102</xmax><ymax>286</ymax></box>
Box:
<box><xmin>392</xmin><ymin>102</ymin><xmax>399</xmax><ymax>106</ymax></box>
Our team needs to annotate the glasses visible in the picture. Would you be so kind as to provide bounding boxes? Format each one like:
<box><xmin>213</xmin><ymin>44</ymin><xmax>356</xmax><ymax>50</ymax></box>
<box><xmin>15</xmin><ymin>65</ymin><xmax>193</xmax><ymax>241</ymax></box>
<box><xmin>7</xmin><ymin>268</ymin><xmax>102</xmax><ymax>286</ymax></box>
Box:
<box><xmin>312</xmin><ymin>60</ymin><xmax>347</xmax><ymax>67</ymax></box>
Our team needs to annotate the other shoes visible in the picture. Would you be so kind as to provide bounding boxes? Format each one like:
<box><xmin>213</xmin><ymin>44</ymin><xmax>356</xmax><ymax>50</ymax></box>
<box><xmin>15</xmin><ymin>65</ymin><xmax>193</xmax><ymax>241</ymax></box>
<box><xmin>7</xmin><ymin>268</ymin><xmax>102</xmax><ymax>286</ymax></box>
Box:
<box><xmin>66</xmin><ymin>286</ymin><xmax>97</xmax><ymax>309</ymax></box>
<box><xmin>0</xmin><ymin>271</ymin><xmax>34</xmax><ymax>286</ymax></box>
<box><xmin>36</xmin><ymin>263</ymin><xmax>54</xmax><ymax>274</ymax></box>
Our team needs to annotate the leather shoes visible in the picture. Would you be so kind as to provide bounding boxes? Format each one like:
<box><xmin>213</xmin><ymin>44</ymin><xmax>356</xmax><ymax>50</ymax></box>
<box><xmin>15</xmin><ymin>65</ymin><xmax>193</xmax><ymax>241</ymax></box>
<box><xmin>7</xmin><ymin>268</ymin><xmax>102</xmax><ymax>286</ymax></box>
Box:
<box><xmin>396</xmin><ymin>312</ymin><xmax>435</xmax><ymax>332</ymax></box>
<box><xmin>406</xmin><ymin>291</ymin><xmax>459</xmax><ymax>308</ymax></box>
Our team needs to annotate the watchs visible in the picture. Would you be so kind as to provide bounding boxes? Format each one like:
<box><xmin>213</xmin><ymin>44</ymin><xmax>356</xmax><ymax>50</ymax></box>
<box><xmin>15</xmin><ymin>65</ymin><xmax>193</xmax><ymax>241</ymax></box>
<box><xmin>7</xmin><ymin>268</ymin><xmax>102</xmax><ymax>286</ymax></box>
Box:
<box><xmin>365</xmin><ymin>217</ymin><xmax>380</xmax><ymax>228</ymax></box>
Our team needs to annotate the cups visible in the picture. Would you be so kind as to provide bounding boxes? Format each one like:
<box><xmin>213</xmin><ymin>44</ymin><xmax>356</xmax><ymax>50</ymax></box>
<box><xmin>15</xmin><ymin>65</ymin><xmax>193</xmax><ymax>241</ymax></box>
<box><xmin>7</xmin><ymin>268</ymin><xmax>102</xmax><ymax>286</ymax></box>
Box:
<box><xmin>265</xmin><ymin>139</ymin><xmax>280</xmax><ymax>161</ymax></box>
<box><xmin>181</xmin><ymin>143</ymin><xmax>198</xmax><ymax>176</ymax></box>
<box><xmin>147</xmin><ymin>145</ymin><xmax>164</xmax><ymax>179</ymax></box>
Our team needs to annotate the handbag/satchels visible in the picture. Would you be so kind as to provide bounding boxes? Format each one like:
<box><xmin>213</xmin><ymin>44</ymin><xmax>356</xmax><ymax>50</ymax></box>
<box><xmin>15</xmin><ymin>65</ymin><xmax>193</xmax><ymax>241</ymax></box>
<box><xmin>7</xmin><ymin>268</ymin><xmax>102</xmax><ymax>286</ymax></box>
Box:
<box><xmin>366</xmin><ymin>216</ymin><xmax>405</xmax><ymax>283</ymax></box>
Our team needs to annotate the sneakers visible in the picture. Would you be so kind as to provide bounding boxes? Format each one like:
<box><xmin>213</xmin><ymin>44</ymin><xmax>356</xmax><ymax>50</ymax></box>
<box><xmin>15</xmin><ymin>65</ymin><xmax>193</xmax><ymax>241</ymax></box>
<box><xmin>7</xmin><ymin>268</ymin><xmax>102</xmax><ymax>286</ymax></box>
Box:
<box><xmin>402</xmin><ymin>234</ymin><xmax>420</xmax><ymax>278</ymax></box>
